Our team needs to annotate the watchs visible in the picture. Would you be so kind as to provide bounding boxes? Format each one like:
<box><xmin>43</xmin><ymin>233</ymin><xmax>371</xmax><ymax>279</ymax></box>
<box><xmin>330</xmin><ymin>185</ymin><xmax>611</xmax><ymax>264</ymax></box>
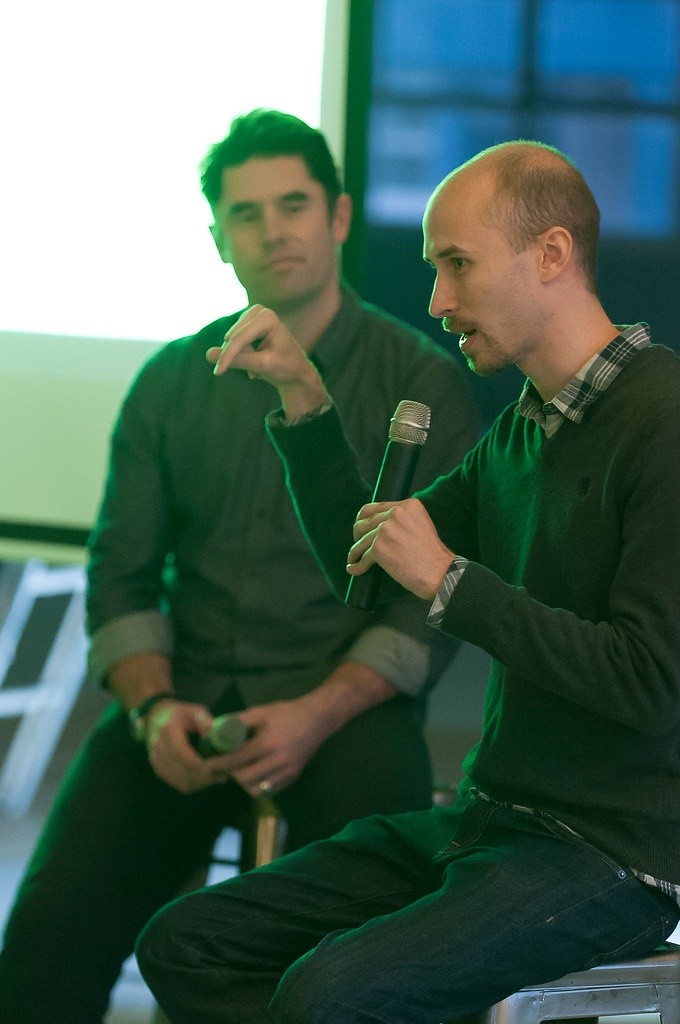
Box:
<box><xmin>126</xmin><ymin>690</ymin><xmax>183</xmax><ymax>745</ymax></box>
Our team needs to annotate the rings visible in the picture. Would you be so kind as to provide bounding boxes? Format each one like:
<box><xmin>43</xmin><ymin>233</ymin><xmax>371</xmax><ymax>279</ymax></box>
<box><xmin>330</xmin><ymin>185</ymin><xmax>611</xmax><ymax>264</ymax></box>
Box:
<box><xmin>258</xmin><ymin>778</ymin><xmax>275</xmax><ymax>795</ymax></box>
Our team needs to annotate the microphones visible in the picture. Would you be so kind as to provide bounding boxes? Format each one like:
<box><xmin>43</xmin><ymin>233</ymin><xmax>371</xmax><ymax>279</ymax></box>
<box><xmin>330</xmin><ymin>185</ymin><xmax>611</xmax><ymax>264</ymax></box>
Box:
<box><xmin>195</xmin><ymin>714</ymin><xmax>247</xmax><ymax>759</ymax></box>
<box><xmin>343</xmin><ymin>400</ymin><xmax>430</xmax><ymax>611</ymax></box>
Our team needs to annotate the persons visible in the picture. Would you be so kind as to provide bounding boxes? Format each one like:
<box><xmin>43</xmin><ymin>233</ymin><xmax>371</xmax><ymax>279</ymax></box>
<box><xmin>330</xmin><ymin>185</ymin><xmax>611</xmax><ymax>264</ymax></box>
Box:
<box><xmin>128</xmin><ymin>140</ymin><xmax>680</xmax><ymax>1024</ymax></box>
<box><xmin>0</xmin><ymin>100</ymin><xmax>491</xmax><ymax>1024</ymax></box>
